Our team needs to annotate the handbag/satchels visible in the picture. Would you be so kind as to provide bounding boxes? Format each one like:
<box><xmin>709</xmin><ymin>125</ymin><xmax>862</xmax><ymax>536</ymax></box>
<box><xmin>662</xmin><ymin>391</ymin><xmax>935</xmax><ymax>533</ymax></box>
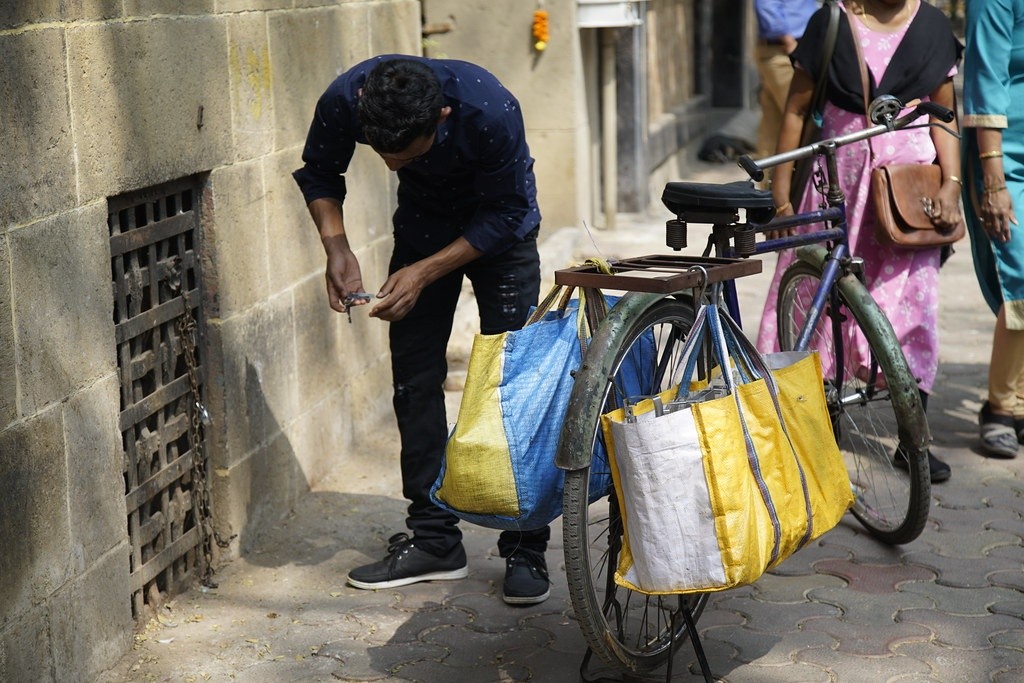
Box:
<box><xmin>870</xmin><ymin>163</ymin><xmax>966</xmax><ymax>247</ymax></box>
<box><xmin>599</xmin><ymin>305</ymin><xmax>856</xmax><ymax>594</ymax></box>
<box><xmin>427</xmin><ymin>255</ymin><xmax>663</xmax><ymax>533</ymax></box>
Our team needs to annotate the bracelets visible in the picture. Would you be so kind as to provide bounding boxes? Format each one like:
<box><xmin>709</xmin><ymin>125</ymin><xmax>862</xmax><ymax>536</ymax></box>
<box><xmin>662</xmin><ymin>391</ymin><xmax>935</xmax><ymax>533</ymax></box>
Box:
<box><xmin>774</xmin><ymin>199</ymin><xmax>790</xmax><ymax>214</ymax></box>
<box><xmin>945</xmin><ymin>176</ymin><xmax>962</xmax><ymax>187</ymax></box>
<box><xmin>980</xmin><ymin>150</ymin><xmax>1005</xmax><ymax>161</ymax></box>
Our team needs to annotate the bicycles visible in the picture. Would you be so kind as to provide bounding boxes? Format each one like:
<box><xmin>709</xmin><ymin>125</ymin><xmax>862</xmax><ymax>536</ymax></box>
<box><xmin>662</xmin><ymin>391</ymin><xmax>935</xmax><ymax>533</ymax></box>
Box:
<box><xmin>553</xmin><ymin>94</ymin><xmax>964</xmax><ymax>681</ymax></box>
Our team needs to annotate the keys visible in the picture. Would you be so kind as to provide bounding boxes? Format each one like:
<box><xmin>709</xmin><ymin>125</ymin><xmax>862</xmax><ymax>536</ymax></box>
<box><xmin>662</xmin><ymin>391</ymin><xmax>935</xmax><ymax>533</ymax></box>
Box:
<box><xmin>340</xmin><ymin>296</ymin><xmax>356</xmax><ymax>325</ymax></box>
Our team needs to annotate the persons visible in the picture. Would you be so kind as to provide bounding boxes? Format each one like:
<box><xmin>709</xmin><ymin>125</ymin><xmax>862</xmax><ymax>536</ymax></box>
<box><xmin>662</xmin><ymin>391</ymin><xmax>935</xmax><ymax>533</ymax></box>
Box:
<box><xmin>753</xmin><ymin>0</ymin><xmax>1024</xmax><ymax>478</ymax></box>
<box><xmin>291</xmin><ymin>53</ymin><xmax>556</xmax><ymax>606</ymax></box>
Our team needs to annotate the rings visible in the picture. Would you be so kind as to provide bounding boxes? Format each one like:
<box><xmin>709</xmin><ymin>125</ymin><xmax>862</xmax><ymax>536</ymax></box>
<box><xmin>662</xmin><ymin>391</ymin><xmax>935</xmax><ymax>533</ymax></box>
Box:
<box><xmin>977</xmin><ymin>216</ymin><xmax>986</xmax><ymax>222</ymax></box>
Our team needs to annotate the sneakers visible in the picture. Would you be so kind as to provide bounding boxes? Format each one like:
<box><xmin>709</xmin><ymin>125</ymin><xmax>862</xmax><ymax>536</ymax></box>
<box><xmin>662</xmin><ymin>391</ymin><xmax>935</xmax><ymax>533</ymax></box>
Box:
<box><xmin>347</xmin><ymin>531</ymin><xmax>468</xmax><ymax>590</ymax></box>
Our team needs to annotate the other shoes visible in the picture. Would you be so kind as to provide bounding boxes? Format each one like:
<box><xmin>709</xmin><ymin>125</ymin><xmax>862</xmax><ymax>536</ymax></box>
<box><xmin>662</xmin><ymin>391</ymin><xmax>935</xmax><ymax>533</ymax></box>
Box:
<box><xmin>502</xmin><ymin>554</ymin><xmax>554</xmax><ymax>605</ymax></box>
<box><xmin>978</xmin><ymin>401</ymin><xmax>1019</xmax><ymax>457</ymax></box>
<box><xmin>893</xmin><ymin>443</ymin><xmax>951</xmax><ymax>483</ymax></box>
